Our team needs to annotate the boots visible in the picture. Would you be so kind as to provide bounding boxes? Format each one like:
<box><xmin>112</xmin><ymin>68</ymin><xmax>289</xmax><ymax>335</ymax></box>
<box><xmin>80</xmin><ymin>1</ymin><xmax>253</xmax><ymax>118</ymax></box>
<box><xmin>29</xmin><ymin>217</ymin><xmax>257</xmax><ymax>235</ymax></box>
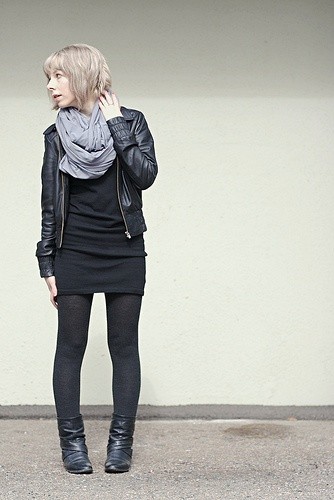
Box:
<box><xmin>56</xmin><ymin>413</ymin><xmax>94</xmax><ymax>473</ymax></box>
<box><xmin>103</xmin><ymin>411</ymin><xmax>137</xmax><ymax>475</ymax></box>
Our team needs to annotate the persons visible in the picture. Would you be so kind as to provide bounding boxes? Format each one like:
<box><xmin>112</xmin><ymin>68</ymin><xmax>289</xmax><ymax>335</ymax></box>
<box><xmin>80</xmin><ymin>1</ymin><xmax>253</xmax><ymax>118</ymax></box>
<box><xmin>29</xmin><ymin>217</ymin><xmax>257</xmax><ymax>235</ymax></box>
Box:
<box><xmin>34</xmin><ymin>41</ymin><xmax>159</xmax><ymax>473</ymax></box>
